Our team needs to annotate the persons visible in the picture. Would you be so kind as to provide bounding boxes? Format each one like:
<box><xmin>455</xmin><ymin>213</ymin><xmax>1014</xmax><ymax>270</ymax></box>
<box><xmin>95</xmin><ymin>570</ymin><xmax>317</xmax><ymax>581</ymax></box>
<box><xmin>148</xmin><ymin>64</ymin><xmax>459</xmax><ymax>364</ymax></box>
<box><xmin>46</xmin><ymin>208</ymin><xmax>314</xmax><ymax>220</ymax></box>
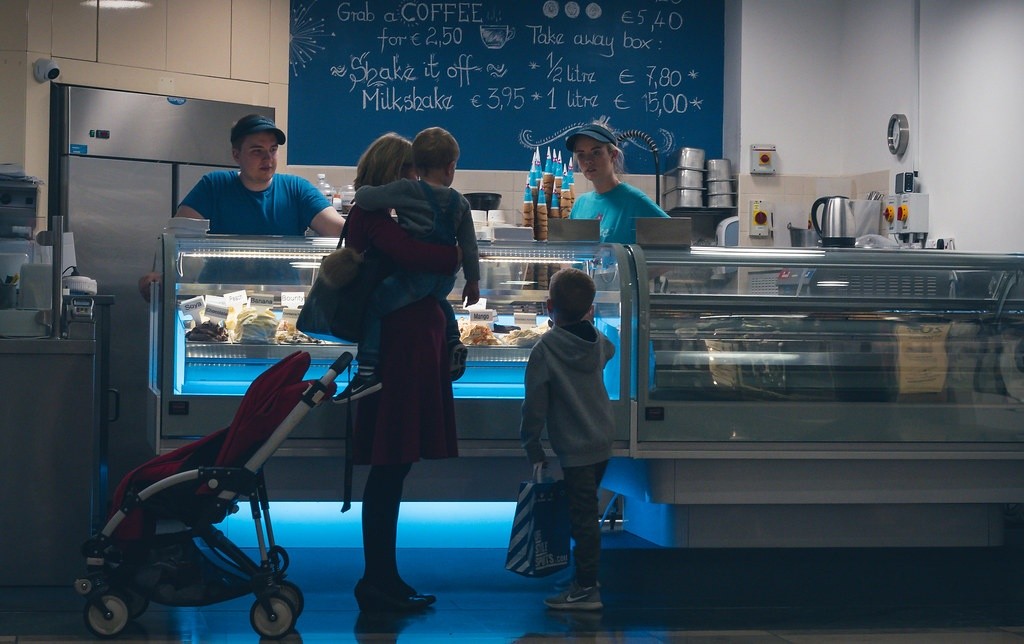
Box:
<box><xmin>333</xmin><ymin>127</ymin><xmax>481</xmax><ymax>403</ymax></box>
<box><xmin>138</xmin><ymin>113</ymin><xmax>347</xmax><ymax>304</ymax></box>
<box><xmin>342</xmin><ymin>132</ymin><xmax>462</xmax><ymax>614</ymax></box>
<box><xmin>565</xmin><ymin>122</ymin><xmax>670</xmax><ymax>330</ymax></box>
<box><xmin>520</xmin><ymin>267</ymin><xmax>615</xmax><ymax>610</ymax></box>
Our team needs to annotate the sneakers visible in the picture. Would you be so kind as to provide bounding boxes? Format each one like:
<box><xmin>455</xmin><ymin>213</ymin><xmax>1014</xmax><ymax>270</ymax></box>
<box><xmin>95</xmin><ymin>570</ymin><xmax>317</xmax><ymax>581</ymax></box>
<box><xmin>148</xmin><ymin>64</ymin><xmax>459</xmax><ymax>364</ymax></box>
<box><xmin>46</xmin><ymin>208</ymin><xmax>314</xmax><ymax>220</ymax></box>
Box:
<box><xmin>544</xmin><ymin>581</ymin><xmax>603</xmax><ymax>608</ymax></box>
<box><xmin>448</xmin><ymin>339</ymin><xmax>468</xmax><ymax>381</ymax></box>
<box><xmin>332</xmin><ymin>373</ymin><xmax>383</xmax><ymax>403</ymax></box>
<box><xmin>554</xmin><ymin>579</ymin><xmax>601</xmax><ymax>590</ymax></box>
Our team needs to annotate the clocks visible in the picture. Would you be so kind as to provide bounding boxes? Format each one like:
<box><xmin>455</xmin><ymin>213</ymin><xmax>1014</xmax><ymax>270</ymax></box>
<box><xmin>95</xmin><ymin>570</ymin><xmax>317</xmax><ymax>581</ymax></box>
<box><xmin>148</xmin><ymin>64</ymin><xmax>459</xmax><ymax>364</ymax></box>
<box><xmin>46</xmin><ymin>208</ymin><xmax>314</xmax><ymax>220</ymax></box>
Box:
<box><xmin>887</xmin><ymin>113</ymin><xmax>909</xmax><ymax>155</ymax></box>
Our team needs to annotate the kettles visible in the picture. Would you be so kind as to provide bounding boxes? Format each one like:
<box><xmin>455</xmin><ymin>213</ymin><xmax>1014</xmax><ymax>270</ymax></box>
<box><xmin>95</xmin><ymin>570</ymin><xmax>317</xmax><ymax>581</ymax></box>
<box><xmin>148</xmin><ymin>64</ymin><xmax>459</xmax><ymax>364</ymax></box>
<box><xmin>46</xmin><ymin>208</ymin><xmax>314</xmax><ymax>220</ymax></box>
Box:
<box><xmin>811</xmin><ymin>196</ymin><xmax>856</xmax><ymax>248</ymax></box>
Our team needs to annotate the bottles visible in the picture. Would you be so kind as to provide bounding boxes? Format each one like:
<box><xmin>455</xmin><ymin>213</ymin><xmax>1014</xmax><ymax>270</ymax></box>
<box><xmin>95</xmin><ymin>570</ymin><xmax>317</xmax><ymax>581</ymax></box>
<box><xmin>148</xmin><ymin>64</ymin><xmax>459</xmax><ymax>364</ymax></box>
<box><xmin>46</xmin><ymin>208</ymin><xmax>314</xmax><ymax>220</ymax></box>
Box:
<box><xmin>340</xmin><ymin>185</ymin><xmax>357</xmax><ymax>214</ymax></box>
<box><xmin>332</xmin><ymin>194</ymin><xmax>342</xmax><ymax>210</ymax></box>
<box><xmin>314</xmin><ymin>173</ymin><xmax>332</xmax><ymax>204</ymax></box>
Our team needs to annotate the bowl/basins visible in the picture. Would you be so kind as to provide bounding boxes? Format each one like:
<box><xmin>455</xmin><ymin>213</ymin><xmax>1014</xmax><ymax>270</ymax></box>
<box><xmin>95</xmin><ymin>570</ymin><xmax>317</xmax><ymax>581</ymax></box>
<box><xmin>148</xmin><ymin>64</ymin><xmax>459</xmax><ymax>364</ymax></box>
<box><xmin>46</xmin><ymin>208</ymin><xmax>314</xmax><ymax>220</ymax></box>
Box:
<box><xmin>463</xmin><ymin>192</ymin><xmax>502</xmax><ymax>211</ymax></box>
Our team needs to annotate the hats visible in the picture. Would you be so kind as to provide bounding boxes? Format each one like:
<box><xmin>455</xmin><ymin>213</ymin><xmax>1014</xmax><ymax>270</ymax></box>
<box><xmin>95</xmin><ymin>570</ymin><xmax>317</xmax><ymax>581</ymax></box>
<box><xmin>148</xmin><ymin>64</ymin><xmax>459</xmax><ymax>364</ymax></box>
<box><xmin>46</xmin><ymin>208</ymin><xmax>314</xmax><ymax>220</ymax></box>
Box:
<box><xmin>565</xmin><ymin>124</ymin><xmax>616</xmax><ymax>152</ymax></box>
<box><xmin>231</xmin><ymin>116</ymin><xmax>286</xmax><ymax>146</ymax></box>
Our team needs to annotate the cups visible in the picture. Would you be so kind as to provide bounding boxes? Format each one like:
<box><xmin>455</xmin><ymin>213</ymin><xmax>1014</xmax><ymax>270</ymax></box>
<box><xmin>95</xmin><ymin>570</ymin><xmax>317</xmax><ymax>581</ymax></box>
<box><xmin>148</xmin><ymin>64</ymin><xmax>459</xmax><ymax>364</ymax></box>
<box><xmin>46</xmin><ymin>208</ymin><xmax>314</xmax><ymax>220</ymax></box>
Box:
<box><xmin>0</xmin><ymin>285</ymin><xmax>17</xmax><ymax>309</ymax></box>
<box><xmin>855</xmin><ymin>200</ymin><xmax>882</xmax><ymax>240</ymax></box>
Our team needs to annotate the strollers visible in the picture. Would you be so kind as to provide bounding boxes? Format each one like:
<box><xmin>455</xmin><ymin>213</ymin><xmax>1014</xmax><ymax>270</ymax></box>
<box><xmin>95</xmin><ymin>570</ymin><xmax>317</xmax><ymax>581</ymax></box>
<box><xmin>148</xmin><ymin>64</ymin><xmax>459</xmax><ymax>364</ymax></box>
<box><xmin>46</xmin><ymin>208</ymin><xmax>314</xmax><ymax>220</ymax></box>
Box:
<box><xmin>78</xmin><ymin>349</ymin><xmax>356</xmax><ymax>641</ymax></box>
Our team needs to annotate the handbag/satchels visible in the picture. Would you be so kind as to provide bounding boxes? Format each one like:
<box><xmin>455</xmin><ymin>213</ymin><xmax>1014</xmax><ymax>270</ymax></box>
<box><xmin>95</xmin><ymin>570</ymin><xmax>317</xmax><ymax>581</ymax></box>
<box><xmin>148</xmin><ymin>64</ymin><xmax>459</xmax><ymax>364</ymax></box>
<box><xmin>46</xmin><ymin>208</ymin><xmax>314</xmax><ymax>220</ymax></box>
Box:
<box><xmin>296</xmin><ymin>205</ymin><xmax>385</xmax><ymax>342</ymax></box>
<box><xmin>505</xmin><ymin>460</ymin><xmax>570</xmax><ymax>577</ymax></box>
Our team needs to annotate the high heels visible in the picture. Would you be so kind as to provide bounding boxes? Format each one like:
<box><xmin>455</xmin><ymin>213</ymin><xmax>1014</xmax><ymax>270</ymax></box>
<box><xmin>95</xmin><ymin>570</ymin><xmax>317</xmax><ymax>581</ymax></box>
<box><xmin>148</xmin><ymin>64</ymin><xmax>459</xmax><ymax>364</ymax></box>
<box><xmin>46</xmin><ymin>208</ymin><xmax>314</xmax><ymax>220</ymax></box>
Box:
<box><xmin>354</xmin><ymin>580</ymin><xmax>428</xmax><ymax>612</ymax></box>
<box><xmin>407</xmin><ymin>591</ymin><xmax>436</xmax><ymax>604</ymax></box>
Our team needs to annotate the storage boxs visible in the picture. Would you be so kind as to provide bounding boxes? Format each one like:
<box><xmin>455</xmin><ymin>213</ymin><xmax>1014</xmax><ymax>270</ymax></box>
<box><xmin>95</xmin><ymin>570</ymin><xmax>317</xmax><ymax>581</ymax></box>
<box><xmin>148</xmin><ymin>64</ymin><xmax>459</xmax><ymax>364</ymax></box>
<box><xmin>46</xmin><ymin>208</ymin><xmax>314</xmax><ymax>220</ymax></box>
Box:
<box><xmin>880</xmin><ymin>322</ymin><xmax>953</xmax><ymax>393</ymax></box>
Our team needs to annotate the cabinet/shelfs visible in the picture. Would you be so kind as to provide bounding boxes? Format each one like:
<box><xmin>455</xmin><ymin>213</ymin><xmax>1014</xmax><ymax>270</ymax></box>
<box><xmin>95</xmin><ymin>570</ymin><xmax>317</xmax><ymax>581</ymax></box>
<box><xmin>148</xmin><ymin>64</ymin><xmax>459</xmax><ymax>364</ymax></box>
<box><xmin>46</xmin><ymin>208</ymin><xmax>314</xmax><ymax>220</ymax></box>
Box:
<box><xmin>163</xmin><ymin>230</ymin><xmax>1024</xmax><ymax>456</ymax></box>
